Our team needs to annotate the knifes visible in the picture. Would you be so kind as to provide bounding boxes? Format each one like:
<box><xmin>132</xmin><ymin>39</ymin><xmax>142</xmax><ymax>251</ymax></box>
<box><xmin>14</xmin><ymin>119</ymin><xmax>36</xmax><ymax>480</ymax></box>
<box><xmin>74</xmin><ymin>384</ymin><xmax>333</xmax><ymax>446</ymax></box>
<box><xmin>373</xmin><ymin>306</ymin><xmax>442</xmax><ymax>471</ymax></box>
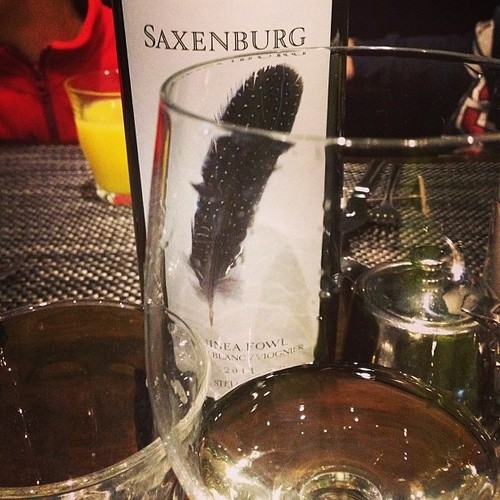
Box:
<box><xmin>338</xmin><ymin>159</ymin><xmax>386</xmax><ymax>236</ymax></box>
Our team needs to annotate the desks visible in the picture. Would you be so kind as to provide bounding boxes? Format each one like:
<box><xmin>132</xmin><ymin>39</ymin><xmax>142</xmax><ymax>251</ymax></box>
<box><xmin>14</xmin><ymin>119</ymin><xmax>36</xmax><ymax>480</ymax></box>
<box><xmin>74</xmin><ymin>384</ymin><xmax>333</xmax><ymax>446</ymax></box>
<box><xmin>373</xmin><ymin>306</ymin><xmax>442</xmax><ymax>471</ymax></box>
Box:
<box><xmin>0</xmin><ymin>140</ymin><xmax>499</xmax><ymax>312</ymax></box>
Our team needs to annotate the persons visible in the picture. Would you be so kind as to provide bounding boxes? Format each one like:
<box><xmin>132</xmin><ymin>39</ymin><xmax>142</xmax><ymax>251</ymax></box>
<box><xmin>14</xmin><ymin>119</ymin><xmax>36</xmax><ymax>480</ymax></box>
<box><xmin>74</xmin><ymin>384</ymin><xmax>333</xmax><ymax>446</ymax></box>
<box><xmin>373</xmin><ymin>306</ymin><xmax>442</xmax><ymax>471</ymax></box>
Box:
<box><xmin>2</xmin><ymin>2</ymin><xmax>120</xmax><ymax>143</ymax></box>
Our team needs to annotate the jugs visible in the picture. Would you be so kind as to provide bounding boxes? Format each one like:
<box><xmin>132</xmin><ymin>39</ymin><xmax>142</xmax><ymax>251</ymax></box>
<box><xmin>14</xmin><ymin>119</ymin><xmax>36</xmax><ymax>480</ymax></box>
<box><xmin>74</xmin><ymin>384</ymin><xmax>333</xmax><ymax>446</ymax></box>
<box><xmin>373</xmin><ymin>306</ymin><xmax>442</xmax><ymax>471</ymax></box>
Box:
<box><xmin>341</xmin><ymin>246</ymin><xmax>499</xmax><ymax>446</ymax></box>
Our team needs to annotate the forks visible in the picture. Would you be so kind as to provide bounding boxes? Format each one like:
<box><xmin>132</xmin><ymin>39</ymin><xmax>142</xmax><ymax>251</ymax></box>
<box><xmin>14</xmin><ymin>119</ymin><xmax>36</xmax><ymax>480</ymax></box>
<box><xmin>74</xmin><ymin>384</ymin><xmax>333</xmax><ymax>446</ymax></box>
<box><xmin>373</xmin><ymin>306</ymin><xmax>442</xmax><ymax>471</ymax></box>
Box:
<box><xmin>362</xmin><ymin>162</ymin><xmax>404</xmax><ymax>241</ymax></box>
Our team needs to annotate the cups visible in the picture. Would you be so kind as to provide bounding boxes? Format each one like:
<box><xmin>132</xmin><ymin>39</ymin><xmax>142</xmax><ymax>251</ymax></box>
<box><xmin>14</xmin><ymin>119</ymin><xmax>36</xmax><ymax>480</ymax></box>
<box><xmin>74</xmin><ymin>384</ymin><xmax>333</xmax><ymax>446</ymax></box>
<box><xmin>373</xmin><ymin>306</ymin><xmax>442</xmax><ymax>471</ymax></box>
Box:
<box><xmin>65</xmin><ymin>68</ymin><xmax>130</xmax><ymax>207</ymax></box>
<box><xmin>141</xmin><ymin>45</ymin><xmax>498</xmax><ymax>499</ymax></box>
<box><xmin>0</xmin><ymin>298</ymin><xmax>211</xmax><ymax>500</ymax></box>
